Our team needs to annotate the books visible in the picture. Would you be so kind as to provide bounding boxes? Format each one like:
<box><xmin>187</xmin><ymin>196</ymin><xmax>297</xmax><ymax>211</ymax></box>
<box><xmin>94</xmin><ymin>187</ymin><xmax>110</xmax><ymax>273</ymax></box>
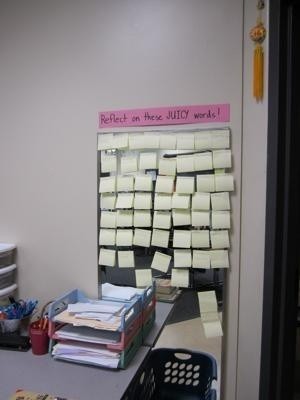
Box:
<box><xmin>53</xmin><ymin>299</ymin><xmax>133</xmax><ymax>370</ymax></box>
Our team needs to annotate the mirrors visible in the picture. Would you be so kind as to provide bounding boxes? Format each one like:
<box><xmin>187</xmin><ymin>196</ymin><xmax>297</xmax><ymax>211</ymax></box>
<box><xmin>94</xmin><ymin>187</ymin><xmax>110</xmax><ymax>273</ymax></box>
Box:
<box><xmin>96</xmin><ymin>126</ymin><xmax>229</xmax><ymax>400</ymax></box>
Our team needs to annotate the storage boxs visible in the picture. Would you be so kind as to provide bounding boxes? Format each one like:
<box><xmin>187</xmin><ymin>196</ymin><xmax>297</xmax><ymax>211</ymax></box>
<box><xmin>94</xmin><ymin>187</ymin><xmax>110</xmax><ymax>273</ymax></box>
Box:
<box><xmin>0</xmin><ymin>244</ymin><xmax>17</xmax><ymax>268</ymax></box>
<box><xmin>0</xmin><ymin>283</ymin><xmax>19</xmax><ymax>312</ymax></box>
<box><xmin>0</xmin><ymin>264</ymin><xmax>17</xmax><ymax>291</ymax></box>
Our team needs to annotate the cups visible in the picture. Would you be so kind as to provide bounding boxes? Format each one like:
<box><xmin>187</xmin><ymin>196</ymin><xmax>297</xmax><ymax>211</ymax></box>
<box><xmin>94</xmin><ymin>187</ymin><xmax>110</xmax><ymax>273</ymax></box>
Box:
<box><xmin>28</xmin><ymin>322</ymin><xmax>50</xmax><ymax>355</ymax></box>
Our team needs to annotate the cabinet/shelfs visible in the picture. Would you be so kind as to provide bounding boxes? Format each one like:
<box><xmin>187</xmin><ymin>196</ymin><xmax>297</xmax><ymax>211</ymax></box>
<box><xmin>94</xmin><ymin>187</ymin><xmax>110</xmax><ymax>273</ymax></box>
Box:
<box><xmin>98</xmin><ymin>282</ymin><xmax>156</xmax><ymax>339</ymax></box>
<box><xmin>50</xmin><ymin>290</ymin><xmax>143</xmax><ymax>369</ymax></box>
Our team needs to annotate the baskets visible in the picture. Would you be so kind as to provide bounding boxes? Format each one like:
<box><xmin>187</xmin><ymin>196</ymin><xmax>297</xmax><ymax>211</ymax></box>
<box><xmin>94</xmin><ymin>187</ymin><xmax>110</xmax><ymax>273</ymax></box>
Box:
<box><xmin>121</xmin><ymin>348</ymin><xmax>217</xmax><ymax>400</ymax></box>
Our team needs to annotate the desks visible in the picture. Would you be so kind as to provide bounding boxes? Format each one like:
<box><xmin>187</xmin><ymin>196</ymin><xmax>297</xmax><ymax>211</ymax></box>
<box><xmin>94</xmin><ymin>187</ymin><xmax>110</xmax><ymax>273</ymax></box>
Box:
<box><xmin>0</xmin><ymin>320</ymin><xmax>151</xmax><ymax>400</ymax></box>
<box><xmin>140</xmin><ymin>289</ymin><xmax>182</xmax><ymax>348</ymax></box>
